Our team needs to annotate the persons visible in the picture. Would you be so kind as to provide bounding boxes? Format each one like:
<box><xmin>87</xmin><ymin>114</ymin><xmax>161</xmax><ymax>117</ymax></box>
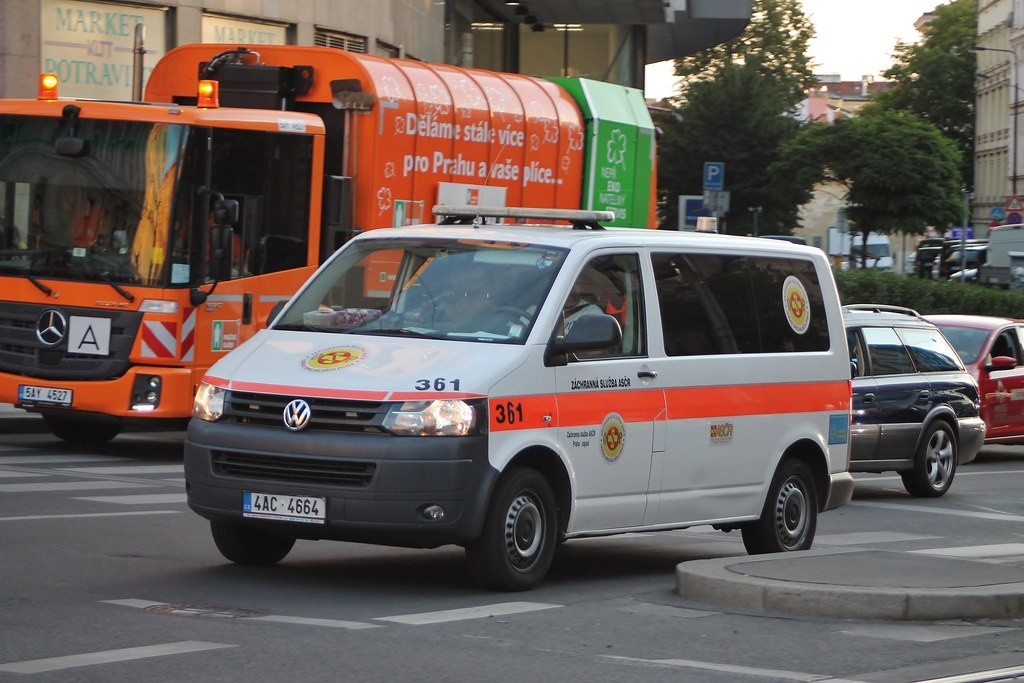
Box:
<box><xmin>57</xmin><ymin>188</ymin><xmax>247</xmax><ymax>269</ymax></box>
<box><xmin>518</xmin><ymin>256</ymin><xmax>607</xmax><ymax>356</ymax></box>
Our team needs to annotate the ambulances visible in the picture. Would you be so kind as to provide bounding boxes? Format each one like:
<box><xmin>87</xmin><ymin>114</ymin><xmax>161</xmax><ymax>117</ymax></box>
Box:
<box><xmin>180</xmin><ymin>204</ymin><xmax>857</xmax><ymax>594</ymax></box>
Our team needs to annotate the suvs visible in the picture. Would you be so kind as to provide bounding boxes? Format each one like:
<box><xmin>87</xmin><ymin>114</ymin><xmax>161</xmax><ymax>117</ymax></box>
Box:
<box><xmin>838</xmin><ymin>301</ymin><xmax>986</xmax><ymax>499</ymax></box>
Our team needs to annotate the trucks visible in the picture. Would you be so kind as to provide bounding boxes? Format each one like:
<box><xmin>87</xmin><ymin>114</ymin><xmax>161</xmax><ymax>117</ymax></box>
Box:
<box><xmin>828</xmin><ymin>226</ymin><xmax>898</xmax><ymax>276</ymax></box>
<box><xmin>0</xmin><ymin>41</ymin><xmax>662</xmax><ymax>450</ymax></box>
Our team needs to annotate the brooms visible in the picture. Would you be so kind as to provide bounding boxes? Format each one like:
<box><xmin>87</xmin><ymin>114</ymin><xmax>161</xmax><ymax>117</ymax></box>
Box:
<box><xmin>334</xmin><ymin>90</ymin><xmax>376</xmax><ymax>240</ymax></box>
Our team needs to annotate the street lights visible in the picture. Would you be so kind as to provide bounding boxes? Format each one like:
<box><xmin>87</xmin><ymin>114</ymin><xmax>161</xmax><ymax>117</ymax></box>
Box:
<box><xmin>967</xmin><ymin>46</ymin><xmax>1019</xmax><ymax>197</ymax></box>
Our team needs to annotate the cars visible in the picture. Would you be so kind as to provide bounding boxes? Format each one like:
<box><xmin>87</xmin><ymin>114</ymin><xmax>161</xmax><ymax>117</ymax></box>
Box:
<box><xmin>919</xmin><ymin>313</ymin><xmax>1024</xmax><ymax>452</ymax></box>
<box><xmin>902</xmin><ymin>236</ymin><xmax>987</xmax><ymax>282</ymax></box>
